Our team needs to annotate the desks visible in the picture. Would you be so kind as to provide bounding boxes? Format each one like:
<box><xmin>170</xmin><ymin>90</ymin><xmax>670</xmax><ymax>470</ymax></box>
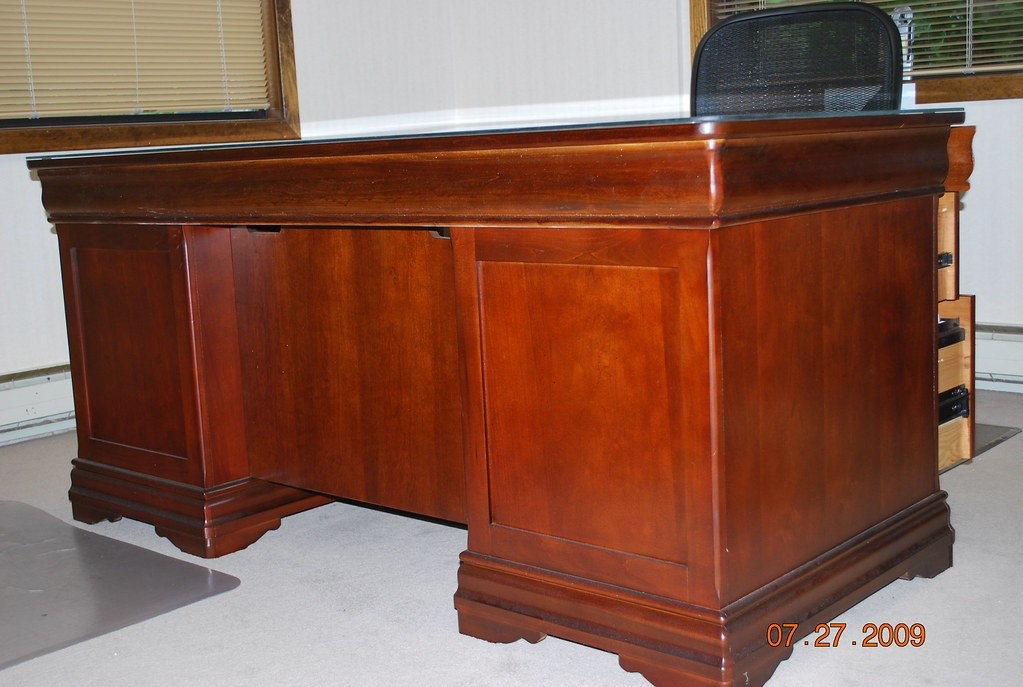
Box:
<box><xmin>26</xmin><ymin>106</ymin><xmax>976</xmax><ymax>687</ymax></box>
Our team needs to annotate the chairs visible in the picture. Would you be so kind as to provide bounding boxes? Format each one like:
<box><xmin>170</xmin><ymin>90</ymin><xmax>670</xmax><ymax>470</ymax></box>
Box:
<box><xmin>689</xmin><ymin>2</ymin><xmax>903</xmax><ymax>117</ymax></box>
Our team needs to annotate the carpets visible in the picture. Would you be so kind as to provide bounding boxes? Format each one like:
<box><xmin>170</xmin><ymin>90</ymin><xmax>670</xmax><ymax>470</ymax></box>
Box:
<box><xmin>0</xmin><ymin>500</ymin><xmax>240</xmax><ymax>672</ymax></box>
